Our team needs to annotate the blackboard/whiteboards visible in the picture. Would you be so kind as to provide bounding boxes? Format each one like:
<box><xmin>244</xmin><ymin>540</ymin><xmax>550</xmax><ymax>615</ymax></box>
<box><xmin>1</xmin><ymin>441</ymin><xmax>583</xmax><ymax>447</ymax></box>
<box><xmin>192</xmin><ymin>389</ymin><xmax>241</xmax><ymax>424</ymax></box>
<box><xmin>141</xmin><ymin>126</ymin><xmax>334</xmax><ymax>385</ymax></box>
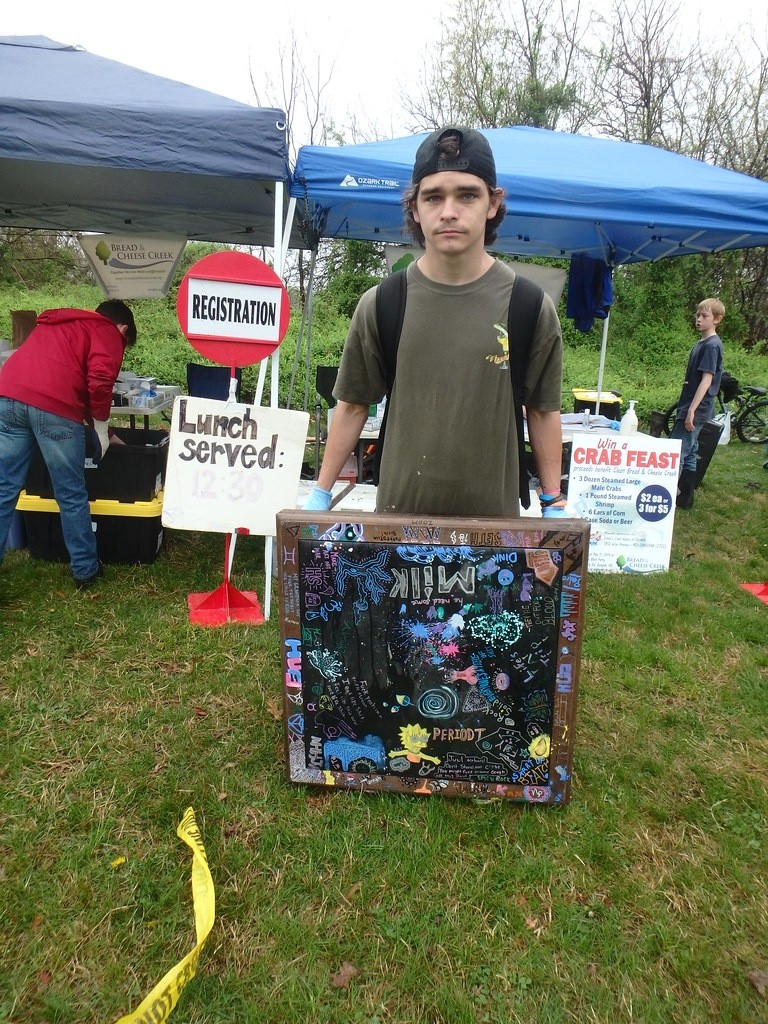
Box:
<box><xmin>274</xmin><ymin>507</ymin><xmax>592</xmax><ymax>805</ymax></box>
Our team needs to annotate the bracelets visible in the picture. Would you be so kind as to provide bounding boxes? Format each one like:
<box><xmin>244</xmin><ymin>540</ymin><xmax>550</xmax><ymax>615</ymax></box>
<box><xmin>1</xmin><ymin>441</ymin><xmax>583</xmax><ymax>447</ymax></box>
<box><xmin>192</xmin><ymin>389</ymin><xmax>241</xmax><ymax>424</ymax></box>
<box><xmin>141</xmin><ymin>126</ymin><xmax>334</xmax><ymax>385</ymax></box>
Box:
<box><xmin>539</xmin><ymin>489</ymin><xmax>566</xmax><ymax>507</ymax></box>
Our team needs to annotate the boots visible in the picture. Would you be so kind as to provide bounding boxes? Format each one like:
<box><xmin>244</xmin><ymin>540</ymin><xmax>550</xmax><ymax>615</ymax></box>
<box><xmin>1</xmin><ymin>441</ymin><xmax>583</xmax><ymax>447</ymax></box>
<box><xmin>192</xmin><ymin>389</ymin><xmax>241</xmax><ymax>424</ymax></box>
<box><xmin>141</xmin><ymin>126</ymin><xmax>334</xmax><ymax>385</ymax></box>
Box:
<box><xmin>676</xmin><ymin>470</ymin><xmax>696</xmax><ymax>509</ymax></box>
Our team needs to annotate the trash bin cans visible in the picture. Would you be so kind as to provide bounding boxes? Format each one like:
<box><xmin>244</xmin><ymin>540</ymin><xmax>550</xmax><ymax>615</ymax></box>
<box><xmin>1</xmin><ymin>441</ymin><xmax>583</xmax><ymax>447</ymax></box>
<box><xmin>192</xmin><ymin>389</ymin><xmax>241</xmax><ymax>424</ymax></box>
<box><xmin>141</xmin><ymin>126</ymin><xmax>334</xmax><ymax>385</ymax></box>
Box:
<box><xmin>572</xmin><ymin>388</ymin><xmax>623</xmax><ymax>421</ymax></box>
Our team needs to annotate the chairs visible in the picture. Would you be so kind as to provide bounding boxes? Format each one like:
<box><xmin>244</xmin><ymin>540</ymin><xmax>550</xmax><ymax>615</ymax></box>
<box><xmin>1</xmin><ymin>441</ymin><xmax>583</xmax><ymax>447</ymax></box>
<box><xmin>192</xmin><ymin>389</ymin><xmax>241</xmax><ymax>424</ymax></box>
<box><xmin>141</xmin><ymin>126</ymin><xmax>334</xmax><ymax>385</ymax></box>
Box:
<box><xmin>161</xmin><ymin>363</ymin><xmax>242</xmax><ymax>424</ymax></box>
<box><xmin>316</xmin><ymin>365</ymin><xmax>339</xmax><ymax>481</ymax></box>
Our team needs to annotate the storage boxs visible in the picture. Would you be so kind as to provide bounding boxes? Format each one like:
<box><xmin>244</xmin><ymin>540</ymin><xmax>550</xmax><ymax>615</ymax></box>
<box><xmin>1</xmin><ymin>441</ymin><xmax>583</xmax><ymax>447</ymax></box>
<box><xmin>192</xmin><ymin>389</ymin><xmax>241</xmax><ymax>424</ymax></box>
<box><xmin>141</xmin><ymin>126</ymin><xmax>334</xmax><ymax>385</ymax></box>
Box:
<box><xmin>114</xmin><ymin>377</ymin><xmax>180</xmax><ymax>408</ymax></box>
<box><xmin>572</xmin><ymin>388</ymin><xmax>623</xmax><ymax>419</ymax></box>
<box><xmin>337</xmin><ymin>456</ymin><xmax>358</xmax><ymax>483</ymax></box>
<box><xmin>4</xmin><ymin>427</ymin><xmax>170</xmax><ymax>564</ymax></box>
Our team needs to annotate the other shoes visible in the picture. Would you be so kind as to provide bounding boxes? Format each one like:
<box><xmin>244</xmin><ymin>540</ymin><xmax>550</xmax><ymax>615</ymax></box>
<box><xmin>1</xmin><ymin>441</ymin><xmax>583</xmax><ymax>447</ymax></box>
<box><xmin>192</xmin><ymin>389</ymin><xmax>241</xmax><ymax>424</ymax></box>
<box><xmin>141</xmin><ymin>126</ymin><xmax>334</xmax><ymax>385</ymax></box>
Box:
<box><xmin>76</xmin><ymin>558</ymin><xmax>103</xmax><ymax>590</ymax></box>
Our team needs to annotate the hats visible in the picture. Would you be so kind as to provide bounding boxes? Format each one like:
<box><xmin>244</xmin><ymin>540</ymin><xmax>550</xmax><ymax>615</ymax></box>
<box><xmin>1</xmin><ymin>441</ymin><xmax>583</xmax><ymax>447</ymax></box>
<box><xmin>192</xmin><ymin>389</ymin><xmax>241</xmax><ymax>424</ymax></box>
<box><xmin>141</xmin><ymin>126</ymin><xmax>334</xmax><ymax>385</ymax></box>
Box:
<box><xmin>411</xmin><ymin>125</ymin><xmax>496</xmax><ymax>188</ymax></box>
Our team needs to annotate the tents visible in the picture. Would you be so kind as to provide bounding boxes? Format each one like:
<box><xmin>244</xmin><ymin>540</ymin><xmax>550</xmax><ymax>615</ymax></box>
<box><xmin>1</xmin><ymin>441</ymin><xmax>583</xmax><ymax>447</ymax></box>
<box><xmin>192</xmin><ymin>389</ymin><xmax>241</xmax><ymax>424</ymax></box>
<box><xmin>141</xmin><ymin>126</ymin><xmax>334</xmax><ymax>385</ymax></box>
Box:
<box><xmin>237</xmin><ymin>125</ymin><xmax>768</xmax><ymax>616</ymax></box>
<box><xmin>0</xmin><ymin>35</ymin><xmax>322</xmax><ymax>628</ymax></box>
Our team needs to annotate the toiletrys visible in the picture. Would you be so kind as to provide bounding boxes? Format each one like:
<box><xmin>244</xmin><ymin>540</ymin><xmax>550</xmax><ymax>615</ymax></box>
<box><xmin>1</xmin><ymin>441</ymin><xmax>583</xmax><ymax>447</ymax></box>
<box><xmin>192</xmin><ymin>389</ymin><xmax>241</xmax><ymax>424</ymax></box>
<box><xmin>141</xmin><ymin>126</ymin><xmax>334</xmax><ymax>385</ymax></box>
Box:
<box><xmin>582</xmin><ymin>408</ymin><xmax>590</xmax><ymax>427</ymax></box>
<box><xmin>620</xmin><ymin>400</ymin><xmax>641</xmax><ymax>434</ymax></box>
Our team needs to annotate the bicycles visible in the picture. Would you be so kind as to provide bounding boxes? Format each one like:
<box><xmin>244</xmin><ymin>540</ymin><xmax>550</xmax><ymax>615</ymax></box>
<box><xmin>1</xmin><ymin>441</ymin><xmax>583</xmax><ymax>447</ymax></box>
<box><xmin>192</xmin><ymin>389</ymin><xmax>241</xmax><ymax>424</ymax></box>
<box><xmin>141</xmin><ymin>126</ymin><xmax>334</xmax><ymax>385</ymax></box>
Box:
<box><xmin>664</xmin><ymin>386</ymin><xmax>768</xmax><ymax>445</ymax></box>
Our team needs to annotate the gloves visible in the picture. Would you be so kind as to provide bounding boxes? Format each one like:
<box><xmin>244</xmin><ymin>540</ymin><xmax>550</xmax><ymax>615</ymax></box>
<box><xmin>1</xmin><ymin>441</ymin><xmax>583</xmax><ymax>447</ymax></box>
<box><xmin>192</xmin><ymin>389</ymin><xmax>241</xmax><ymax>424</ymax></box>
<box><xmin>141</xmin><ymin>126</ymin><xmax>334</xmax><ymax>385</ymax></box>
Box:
<box><xmin>304</xmin><ymin>487</ymin><xmax>332</xmax><ymax>511</ymax></box>
<box><xmin>90</xmin><ymin>417</ymin><xmax>110</xmax><ymax>465</ymax></box>
<box><xmin>542</xmin><ymin>507</ymin><xmax>568</xmax><ymax>518</ymax></box>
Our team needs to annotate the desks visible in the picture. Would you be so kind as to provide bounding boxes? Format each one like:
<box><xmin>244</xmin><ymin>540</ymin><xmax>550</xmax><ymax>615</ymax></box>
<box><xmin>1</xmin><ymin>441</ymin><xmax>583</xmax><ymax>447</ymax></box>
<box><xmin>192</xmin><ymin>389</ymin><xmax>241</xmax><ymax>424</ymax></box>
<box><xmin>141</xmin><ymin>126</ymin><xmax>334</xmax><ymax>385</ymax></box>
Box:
<box><xmin>358</xmin><ymin>415</ymin><xmax>658</xmax><ymax>484</ymax></box>
<box><xmin>110</xmin><ymin>385</ymin><xmax>181</xmax><ymax>429</ymax></box>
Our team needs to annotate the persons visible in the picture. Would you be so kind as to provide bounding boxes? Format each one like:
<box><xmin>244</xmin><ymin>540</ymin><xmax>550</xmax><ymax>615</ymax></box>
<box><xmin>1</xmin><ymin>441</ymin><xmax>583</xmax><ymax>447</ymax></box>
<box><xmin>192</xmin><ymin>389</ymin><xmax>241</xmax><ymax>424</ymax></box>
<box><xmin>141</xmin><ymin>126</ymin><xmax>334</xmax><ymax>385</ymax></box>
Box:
<box><xmin>669</xmin><ymin>298</ymin><xmax>725</xmax><ymax>509</ymax></box>
<box><xmin>1</xmin><ymin>299</ymin><xmax>137</xmax><ymax>591</ymax></box>
<box><xmin>300</xmin><ymin>124</ymin><xmax>584</xmax><ymax>518</ymax></box>
